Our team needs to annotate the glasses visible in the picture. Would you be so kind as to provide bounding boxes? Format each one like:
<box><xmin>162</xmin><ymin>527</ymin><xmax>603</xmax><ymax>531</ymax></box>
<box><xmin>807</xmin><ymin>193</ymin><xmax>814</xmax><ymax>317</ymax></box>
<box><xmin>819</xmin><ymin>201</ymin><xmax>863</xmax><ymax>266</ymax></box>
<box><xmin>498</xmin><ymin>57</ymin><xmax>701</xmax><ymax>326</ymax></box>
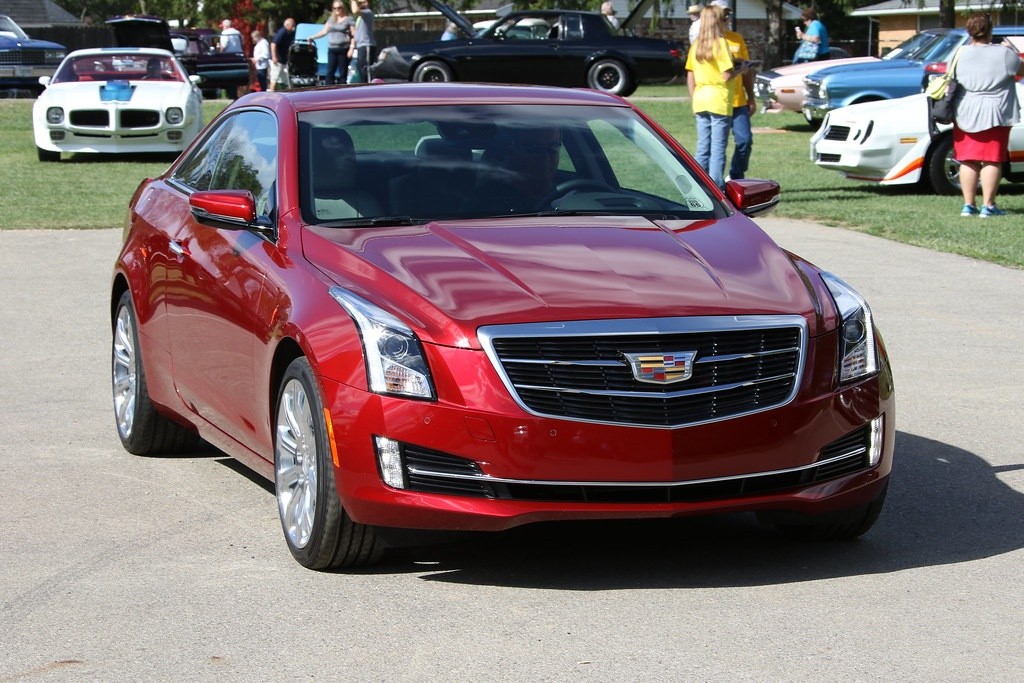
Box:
<box><xmin>334</xmin><ymin>7</ymin><xmax>341</xmax><ymax>10</ymax></box>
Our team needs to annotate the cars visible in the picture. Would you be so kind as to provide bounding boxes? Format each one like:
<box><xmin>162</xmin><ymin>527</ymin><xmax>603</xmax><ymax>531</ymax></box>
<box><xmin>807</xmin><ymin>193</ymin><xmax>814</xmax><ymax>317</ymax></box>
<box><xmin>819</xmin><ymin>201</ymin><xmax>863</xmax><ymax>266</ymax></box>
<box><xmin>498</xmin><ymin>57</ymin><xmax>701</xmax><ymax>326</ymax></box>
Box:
<box><xmin>32</xmin><ymin>45</ymin><xmax>204</xmax><ymax>164</ymax></box>
<box><xmin>751</xmin><ymin>54</ymin><xmax>881</xmax><ymax>115</ymax></box>
<box><xmin>810</xmin><ymin>37</ymin><xmax>1024</xmax><ymax>194</ymax></box>
<box><xmin>367</xmin><ymin>0</ymin><xmax>687</xmax><ymax>98</ymax></box>
<box><xmin>106</xmin><ymin>15</ymin><xmax>251</xmax><ymax>98</ymax></box>
<box><xmin>803</xmin><ymin>26</ymin><xmax>1024</xmax><ymax>128</ymax></box>
<box><xmin>110</xmin><ymin>81</ymin><xmax>894</xmax><ymax>575</ymax></box>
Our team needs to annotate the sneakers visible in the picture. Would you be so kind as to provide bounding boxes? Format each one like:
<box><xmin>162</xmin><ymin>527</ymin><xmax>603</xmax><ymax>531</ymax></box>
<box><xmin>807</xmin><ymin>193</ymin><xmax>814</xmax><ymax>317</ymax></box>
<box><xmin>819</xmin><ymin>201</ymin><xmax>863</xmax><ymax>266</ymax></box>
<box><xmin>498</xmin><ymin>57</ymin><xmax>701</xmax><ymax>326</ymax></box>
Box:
<box><xmin>978</xmin><ymin>203</ymin><xmax>1006</xmax><ymax>218</ymax></box>
<box><xmin>960</xmin><ymin>204</ymin><xmax>980</xmax><ymax>217</ymax></box>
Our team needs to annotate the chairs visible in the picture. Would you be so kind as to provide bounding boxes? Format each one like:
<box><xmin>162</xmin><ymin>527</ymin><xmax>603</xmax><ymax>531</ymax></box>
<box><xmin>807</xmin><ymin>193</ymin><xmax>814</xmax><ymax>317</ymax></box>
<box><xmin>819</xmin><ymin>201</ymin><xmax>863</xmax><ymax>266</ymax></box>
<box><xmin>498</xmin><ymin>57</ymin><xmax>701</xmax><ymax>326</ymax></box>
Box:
<box><xmin>474</xmin><ymin>146</ymin><xmax>523</xmax><ymax>213</ymax></box>
<box><xmin>309</xmin><ymin>127</ymin><xmax>382</xmax><ymax>221</ymax></box>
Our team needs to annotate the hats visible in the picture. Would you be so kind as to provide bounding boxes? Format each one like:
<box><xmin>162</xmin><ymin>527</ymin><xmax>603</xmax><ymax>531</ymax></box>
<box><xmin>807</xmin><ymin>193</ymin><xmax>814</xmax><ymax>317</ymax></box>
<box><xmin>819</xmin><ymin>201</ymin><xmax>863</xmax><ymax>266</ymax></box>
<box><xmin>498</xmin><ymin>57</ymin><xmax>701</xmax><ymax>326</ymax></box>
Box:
<box><xmin>711</xmin><ymin>0</ymin><xmax>731</xmax><ymax>11</ymax></box>
<box><xmin>687</xmin><ymin>5</ymin><xmax>702</xmax><ymax>14</ymax></box>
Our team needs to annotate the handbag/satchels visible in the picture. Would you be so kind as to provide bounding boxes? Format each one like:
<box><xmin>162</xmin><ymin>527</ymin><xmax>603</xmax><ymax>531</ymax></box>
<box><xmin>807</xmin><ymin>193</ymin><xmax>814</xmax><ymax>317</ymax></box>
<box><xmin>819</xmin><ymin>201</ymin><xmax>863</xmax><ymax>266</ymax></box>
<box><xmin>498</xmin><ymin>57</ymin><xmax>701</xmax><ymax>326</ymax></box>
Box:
<box><xmin>798</xmin><ymin>42</ymin><xmax>818</xmax><ymax>60</ymax></box>
<box><xmin>926</xmin><ymin>77</ymin><xmax>946</xmax><ymax>100</ymax></box>
<box><xmin>930</xmin><ymin>79</ymin><xmax>958</xmax><ymax>125</ymax></box>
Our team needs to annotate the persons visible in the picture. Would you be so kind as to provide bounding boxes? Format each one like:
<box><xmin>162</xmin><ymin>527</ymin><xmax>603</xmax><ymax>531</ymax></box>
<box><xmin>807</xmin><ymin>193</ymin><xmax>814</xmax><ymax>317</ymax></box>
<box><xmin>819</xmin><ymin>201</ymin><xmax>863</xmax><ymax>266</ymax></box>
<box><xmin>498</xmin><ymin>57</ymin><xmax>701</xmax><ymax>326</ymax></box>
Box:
<box><xmin>952</xmin><ymin>14</ymin><xmax>1024</xmax><ymax>220</ymax></box>
<box><xmin>601</xmin><ymin>2</ymin><xmax>622</xmax><ymax>33</ymax></box>
<box><xmin>249</xmin><ymin>31</ymin><xmax>270</xmax><ymax>91</ymax></box>
<box><xmin>306</xmin><ymin>0</ymin><xmax>376</xmax><ymax>89</ymax></box>
<box><xmin>482</xmin><ymin>121</ymin><xmax>572</xmax><ymax>215</ymax></box>
<box><xmin>221</xmin><ymin>19</ymin><xmax>245</xmax><ymax>100</ymax></box>
<box><xmin>268</xmin><ymin>18</ymin><xmax>295</xmax><ymax>91</ymax></box>
<box><xmin>791</xmin><ymin>9</ymin><xmax>831</xmax><ymax>65</ymax></box>
<box><xmin>139</xmin><ymin>58</ymin><xmax>175</xmax><ymax>79</ymax></box>
<box><xmin>441</xmin><ymin>23</ymin><xmax>459</xmax><ymax>41</ymax></box>
<box><xmin>685</xmin><ymin>0</ymin><xmax>756</xmax><ymax>190</ymax></box>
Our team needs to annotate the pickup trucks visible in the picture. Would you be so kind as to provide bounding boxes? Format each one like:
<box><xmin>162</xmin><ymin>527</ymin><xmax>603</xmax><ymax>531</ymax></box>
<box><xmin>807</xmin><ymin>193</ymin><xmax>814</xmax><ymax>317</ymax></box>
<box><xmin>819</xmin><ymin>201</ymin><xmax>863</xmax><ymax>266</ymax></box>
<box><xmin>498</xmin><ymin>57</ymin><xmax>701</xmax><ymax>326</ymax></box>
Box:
<box><xmin>0</xmin><ymin>12</ymin><xmax>66</xmax><ymax>99</ymax></box>
